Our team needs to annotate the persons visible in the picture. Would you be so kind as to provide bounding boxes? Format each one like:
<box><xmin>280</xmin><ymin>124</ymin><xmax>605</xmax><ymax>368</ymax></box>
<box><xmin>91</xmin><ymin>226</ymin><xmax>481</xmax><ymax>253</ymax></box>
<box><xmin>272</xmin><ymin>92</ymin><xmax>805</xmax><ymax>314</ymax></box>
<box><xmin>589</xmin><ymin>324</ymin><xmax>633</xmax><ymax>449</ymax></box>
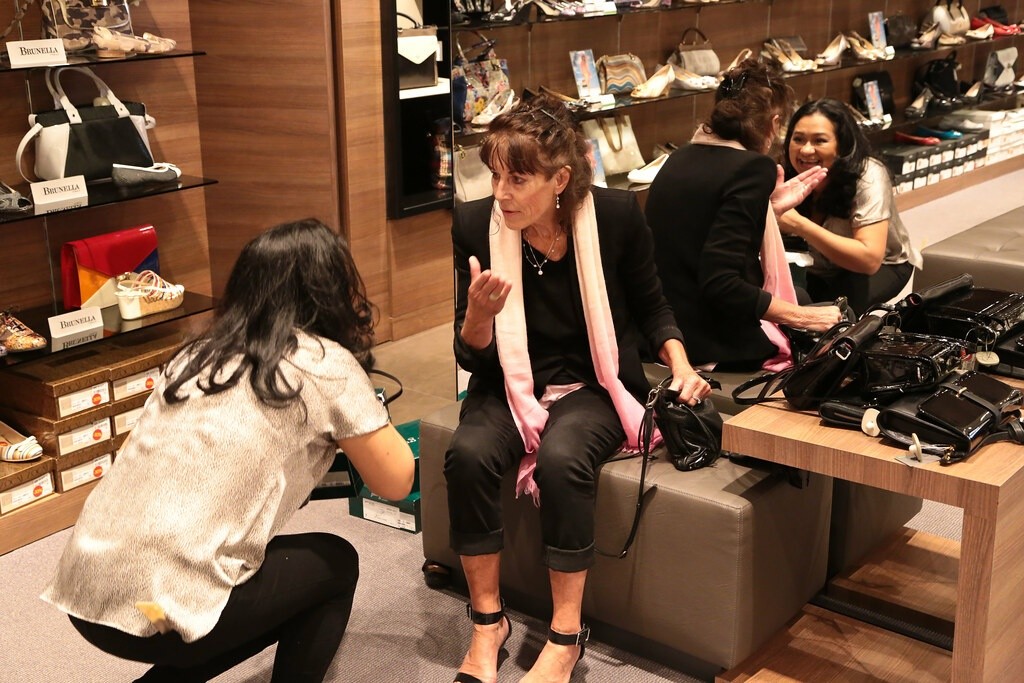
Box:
<box><xmin>644</xmin><ymin>58</ymin><xmax>844</xmax><ymax>377</ymax></box>
<box><xmin>40</xmin><ymin>215</ymin><xmax>415</xmax><ymax>683</ymax></box>
<box><xmin>774</xmin><ymin>99</ymin><xmax>924</xmax><ymax>306</ymax></box>
<box><xmin>580</xmin><ymin>55</ymin><xmax>591</xmax><ymax>88</ymax></box>
<box><xmin>444</xmin><ymin>90</ymin><xmax>713</xmax><ymax>683</ymax></box>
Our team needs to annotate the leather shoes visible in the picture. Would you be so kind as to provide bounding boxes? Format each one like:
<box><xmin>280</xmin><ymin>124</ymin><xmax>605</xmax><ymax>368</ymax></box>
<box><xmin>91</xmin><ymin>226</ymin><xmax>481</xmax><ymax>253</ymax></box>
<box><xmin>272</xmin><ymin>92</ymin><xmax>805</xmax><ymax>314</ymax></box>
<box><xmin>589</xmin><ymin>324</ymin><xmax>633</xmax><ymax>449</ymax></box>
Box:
<box><xmin>754</xmin><ymin>0</ymin><xmax>1024</xmax><ymax>148</ymax></box>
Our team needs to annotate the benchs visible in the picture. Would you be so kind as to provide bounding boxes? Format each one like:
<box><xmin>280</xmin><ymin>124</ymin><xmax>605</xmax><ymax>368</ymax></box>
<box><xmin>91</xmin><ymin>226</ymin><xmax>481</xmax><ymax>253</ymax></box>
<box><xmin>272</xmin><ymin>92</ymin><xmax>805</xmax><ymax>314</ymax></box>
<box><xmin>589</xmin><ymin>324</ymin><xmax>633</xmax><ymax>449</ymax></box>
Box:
<box><xmin>418</xmin><ymin>208</ymin><xmax>1024</xmax><ymax>683</ymax></box>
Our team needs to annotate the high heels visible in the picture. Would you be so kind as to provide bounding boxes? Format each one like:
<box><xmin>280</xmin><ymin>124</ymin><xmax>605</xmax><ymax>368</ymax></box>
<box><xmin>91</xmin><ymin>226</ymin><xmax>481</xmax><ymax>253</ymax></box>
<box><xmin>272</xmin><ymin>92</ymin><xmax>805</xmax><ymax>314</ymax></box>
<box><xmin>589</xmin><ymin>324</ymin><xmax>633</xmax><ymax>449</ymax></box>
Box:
<box><xmin>469</xmin><ymin>88</ymin><xmax>522</xmax><ymax>127</ymax></box>
<box><xmin>113</xmin><ymin>269</ymin><xmax>186</xmax><ymax>320</ymax></box>
<box><xmin>629</xmin><ymin>61</ymin><xmax>722</xmax><ymax>100</ymax></box>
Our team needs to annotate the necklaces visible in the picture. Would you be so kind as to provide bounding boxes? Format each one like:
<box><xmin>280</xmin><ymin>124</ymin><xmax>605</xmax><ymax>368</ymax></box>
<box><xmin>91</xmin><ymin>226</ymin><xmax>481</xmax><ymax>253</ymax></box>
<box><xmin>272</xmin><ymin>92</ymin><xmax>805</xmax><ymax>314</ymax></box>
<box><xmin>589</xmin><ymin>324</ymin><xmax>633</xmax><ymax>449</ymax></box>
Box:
<box><xmin>522</xmin><ymin>227</ymin><xmax>562</xmax><ymax>275</ymax></box>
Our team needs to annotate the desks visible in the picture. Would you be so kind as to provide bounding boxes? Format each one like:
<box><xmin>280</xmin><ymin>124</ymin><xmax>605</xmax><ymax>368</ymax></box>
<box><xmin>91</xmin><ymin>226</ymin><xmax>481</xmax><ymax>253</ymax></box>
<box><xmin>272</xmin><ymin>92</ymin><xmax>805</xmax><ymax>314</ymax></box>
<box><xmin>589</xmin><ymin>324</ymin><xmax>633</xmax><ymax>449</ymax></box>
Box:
<box><xmin>722</xmin><ymin>375</ymin><xmax>1024</xmax><ymax>683</ymax></box>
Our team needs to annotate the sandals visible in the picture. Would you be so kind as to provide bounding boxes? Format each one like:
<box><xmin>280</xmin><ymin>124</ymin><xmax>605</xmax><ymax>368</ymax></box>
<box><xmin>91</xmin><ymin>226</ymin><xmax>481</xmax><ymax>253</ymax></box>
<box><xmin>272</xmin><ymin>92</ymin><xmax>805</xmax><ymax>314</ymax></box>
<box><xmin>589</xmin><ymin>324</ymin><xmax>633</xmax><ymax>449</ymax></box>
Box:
<box><xmin>451</xmin><ymin>597</ymin><xmax>514</xmax><ymax>683</ymax></box>
<box><xmin>516</xmin><ymin>618</ymin><xmax>593</xmax><ymax>683</ymax></box>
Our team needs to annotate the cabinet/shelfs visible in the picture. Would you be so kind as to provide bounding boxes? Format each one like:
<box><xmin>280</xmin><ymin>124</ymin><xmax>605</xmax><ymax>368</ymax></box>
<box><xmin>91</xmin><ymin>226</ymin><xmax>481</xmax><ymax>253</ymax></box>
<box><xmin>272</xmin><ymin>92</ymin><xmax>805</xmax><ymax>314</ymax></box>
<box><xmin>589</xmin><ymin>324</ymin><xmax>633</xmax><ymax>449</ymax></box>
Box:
<box><xmin>0</xmin><ymin>49</ymin><xmax>227</xmax><ymax>366</ymax></box>
<box><xmin>453</xmin><ymin>0</ymin><xmax>1024</xmax><ymax>145</ymax></box>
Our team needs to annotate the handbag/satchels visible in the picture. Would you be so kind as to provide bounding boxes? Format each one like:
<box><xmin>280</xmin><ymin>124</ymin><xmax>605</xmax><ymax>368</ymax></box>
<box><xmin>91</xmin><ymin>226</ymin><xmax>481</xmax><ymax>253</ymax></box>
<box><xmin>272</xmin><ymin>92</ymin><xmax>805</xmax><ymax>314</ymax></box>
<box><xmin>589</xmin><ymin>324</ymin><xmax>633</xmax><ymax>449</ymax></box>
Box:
<box><xmin>731</xmin><ymin>271</ymin><xmax>1024</xmax><ymax>437</ymax></box>
<box><xmin>59</xmin><ymin>222</ymin><xmax>161</xmax><ymax>311</ymax></box>
<box><xmin>15</xmin><ymin>66</ymin><xmax>157</xmax><ymax>191</ymax></box>
<box><xmin>678</xmin><ymin>27</ymin><xmax>719</xmax><ymax>76</ymax></box>
<box><xmin>594</xmin><ymin>51</ymin><xmax>648</xmax><ymax>96</ymax></box>
<box><xmin>644</xmin><ymin>365</ymin><xmax>722</xmax><ymax>472</ymax></box>
<box><xmin>578</xmin><ymin>109</ymin><xmax>647</xmax><ymax>178</ymax></box>
<box><xmin>397</xmin><ymin>11</ymin><xmax>439</xmax><ymax>91</ymax></box>
<box><xmin>452</xmin><ymin>29</ymin><xmax>513</xmax><ymax>125</ymax></box>
<box><xmin>40</xmin><ymin>0</ymin><xmax>135</xmax><ymax>58</ymax></box>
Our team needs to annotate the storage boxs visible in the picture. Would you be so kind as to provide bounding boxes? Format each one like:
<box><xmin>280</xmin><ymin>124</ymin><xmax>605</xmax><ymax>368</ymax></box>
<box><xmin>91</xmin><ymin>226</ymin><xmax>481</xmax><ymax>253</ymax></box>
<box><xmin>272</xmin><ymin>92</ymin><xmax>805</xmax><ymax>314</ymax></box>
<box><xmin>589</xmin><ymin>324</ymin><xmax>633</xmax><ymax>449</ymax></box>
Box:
<box><xmin>306</xmin><ymin>416</ymin><xmax>428</xmax><ymax>533</ymax></box>
<box><xmin>0</xmin><ymin>333</ymin><xmax>197</xmax><ymax>522</ymax></box>
<box><xmin>873</xmin><ymin>105</ymin><xmax>1023</xmax><ymax>197</ymax></box>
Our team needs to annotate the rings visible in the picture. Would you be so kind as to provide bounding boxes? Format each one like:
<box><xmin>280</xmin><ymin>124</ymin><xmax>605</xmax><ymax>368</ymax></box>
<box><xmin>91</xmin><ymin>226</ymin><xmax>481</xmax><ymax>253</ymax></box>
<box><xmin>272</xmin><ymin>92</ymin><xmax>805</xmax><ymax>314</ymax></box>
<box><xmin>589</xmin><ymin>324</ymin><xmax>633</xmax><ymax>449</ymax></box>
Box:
<box><xmin>692</xmin><ymin>397</ymin><xmax>700</xmax><ymax>403</ymax></box>
<box><xmin>840</xmin><ymin>320</ymin><xmax>844</xmax><ymax>322</ymax></box>
<box><xmin>490</xmin><ymin>295</ymin><xmax>498</xmax><ymax>300</ymax></box>
<box><xmin>804</xmin><ymin>186</ymin><xmax>807</xmax><ymax>189</ymax></box>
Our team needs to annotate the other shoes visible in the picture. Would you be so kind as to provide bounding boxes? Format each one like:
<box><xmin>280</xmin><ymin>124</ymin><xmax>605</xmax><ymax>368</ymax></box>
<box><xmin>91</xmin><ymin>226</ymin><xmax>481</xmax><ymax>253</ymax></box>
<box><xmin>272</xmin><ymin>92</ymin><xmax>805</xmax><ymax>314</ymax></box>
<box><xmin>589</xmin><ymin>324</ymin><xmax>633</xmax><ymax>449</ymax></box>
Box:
<box><xmin>0</xmin><ymin>420</ymin><xmax>44</xmax><ymax>462</ymax></box>
<box><xmin>91</xmin><ymin>24</ymin><xmax>177</xmax><ymax>59</ymax></box>
<box><xmin>0</xmin><ymin>178</ymin><xmax>34</xmax><ymax>213</ymax></box>
<box><xmin>0</xmin><ymin>305</ymin><xmax>48</xmax><ymax>353</ymax></box>
<box><xmin>626</xmin><ymin>141</ymin><xmax>678</xmax><ymax>184</ymax></box>
<box><xmin>111</xmin><ymin>161</ymin><xmax>182</xmax><ymax>190</ymax></box>
<box><xmin>521</xmin><ymin>85</ymin><xmax>588</xmax><ymax>113</ymax></box>
<box><xmin>481</xmin><ymin>0</ymin><xmax>671</xmax><ymax>22</ymax></box>
